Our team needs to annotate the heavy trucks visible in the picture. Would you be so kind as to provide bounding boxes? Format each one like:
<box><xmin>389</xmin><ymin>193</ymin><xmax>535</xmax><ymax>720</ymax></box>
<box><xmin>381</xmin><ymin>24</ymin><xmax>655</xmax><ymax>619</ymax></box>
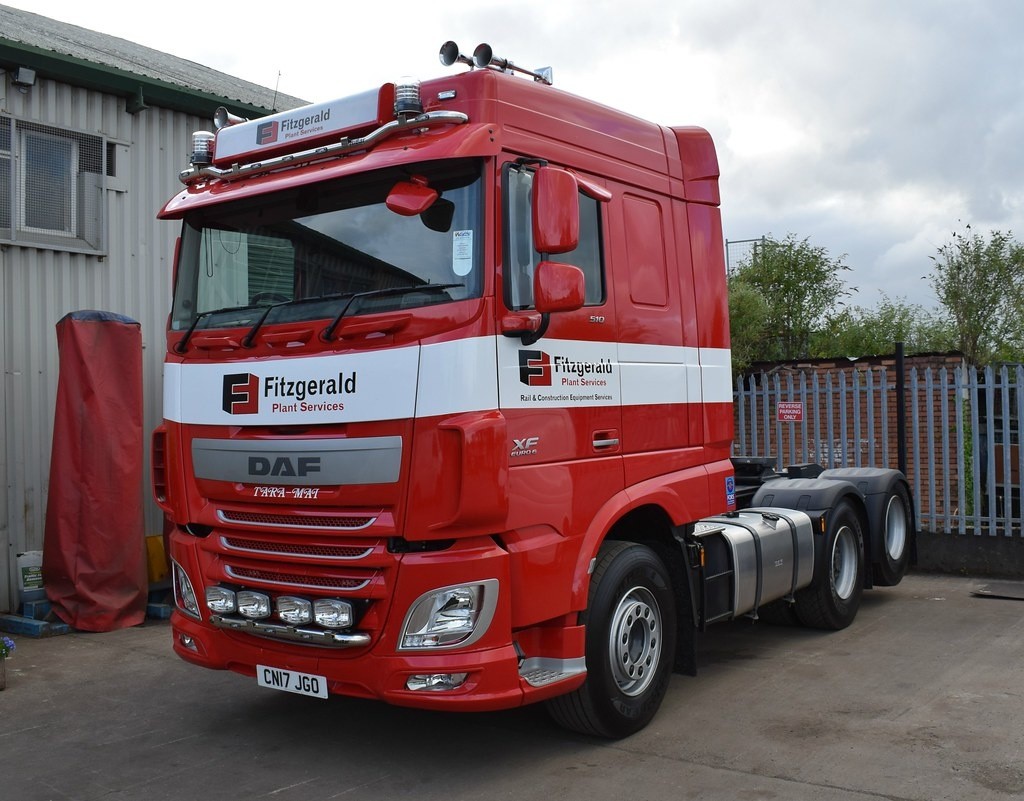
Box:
<box><xmin>150</xmin><ymin>39</ymin><xmax>916</xmax><ymax>741</ymax></box>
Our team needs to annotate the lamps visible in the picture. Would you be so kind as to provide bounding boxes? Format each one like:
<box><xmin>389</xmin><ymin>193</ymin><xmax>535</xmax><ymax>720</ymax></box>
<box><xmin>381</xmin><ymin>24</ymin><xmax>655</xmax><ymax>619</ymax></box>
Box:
<box><xmin>12</xmin><ymin>66</ymin><xmax>37</xmax><ymax>95</ymax></box>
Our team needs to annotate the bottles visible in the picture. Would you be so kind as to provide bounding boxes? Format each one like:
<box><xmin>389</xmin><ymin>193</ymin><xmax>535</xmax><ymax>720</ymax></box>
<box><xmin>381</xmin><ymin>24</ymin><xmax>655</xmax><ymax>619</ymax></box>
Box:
<box><xmin>17</xmin><ymin>551</ymin><xmax>43</xmax><ymax>591</ymax></box>
